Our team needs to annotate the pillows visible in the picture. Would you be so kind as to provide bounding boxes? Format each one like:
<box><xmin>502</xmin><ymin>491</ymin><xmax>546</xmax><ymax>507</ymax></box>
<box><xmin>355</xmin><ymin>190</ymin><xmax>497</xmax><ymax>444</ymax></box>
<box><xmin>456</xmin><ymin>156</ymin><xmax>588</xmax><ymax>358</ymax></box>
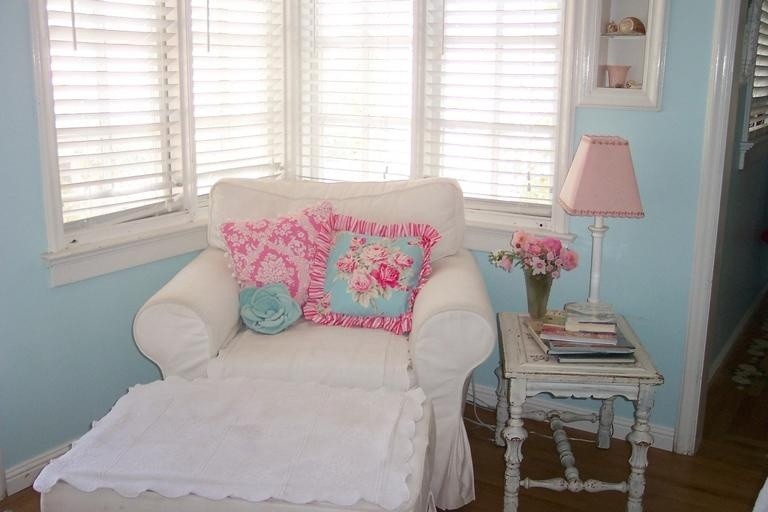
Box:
<box><xmin>219</xmin><ymin>200</ymin><xmax>442</xmax><ymax>336</ymax></box>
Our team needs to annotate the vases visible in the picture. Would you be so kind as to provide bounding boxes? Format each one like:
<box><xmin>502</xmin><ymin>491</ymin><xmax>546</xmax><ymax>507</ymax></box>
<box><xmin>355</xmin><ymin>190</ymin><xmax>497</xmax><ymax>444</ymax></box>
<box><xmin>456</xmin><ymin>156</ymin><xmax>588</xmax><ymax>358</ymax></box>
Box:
<box><xmin>522</xmin><ymin>270</ymin><xmax>553</xmax><ymax>320</ymax></box>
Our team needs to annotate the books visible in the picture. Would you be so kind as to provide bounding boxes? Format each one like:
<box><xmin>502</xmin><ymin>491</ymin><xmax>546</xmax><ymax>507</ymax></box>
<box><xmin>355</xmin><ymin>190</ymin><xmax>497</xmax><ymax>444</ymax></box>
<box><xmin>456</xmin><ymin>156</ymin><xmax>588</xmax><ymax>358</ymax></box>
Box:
<box><xmin>522</xmin><ymin>303</ymin><xmax>637</xmax><ymax>367</ymax></box>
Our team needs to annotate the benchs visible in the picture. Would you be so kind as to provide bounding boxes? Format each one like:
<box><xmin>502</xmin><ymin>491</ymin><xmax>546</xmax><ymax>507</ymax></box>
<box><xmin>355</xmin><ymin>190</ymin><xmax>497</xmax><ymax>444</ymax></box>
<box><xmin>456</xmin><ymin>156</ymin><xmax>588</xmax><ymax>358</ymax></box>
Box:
<box><xmin>33</xmin><ymin>376</ymin><xmax>436</xmax><ymax>512</ymax></box>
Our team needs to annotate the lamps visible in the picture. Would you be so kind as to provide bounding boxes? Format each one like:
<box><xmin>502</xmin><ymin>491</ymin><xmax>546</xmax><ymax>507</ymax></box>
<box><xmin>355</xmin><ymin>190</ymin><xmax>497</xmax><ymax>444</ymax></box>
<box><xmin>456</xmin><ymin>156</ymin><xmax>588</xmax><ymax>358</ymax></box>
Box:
<box><xmin>557</xmin><ymin>135</ymin><xmax>644</xmax><ymax>316</ymax></box>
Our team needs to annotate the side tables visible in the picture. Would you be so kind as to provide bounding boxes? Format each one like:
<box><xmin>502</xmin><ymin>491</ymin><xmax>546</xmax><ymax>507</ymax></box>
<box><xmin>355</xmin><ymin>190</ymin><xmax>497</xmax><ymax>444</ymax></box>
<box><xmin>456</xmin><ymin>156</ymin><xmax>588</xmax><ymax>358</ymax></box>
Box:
<box><xmin>495</xmin><ymin>311</ymin><xmax>664</xmax><ymax>512</ymax></box>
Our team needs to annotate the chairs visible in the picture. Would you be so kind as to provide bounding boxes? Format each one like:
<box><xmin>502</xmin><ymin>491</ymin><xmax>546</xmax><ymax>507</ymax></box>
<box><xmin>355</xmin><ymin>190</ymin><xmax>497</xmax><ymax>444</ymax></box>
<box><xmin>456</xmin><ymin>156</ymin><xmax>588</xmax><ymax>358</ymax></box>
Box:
<box><xmin>133</xmin><ymin>177</ymin><xmax>496</xmax><ymax>512</ymax></box>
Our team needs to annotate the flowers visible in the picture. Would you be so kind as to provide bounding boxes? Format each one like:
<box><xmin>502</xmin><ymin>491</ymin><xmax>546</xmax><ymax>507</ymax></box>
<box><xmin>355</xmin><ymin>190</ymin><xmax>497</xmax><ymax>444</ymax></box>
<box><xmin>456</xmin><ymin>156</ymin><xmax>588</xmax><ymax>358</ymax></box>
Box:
<box><xmin>489</xmin><ymin>229</ymin><xmax>578</xmax><ymax>280</ymax></box>
